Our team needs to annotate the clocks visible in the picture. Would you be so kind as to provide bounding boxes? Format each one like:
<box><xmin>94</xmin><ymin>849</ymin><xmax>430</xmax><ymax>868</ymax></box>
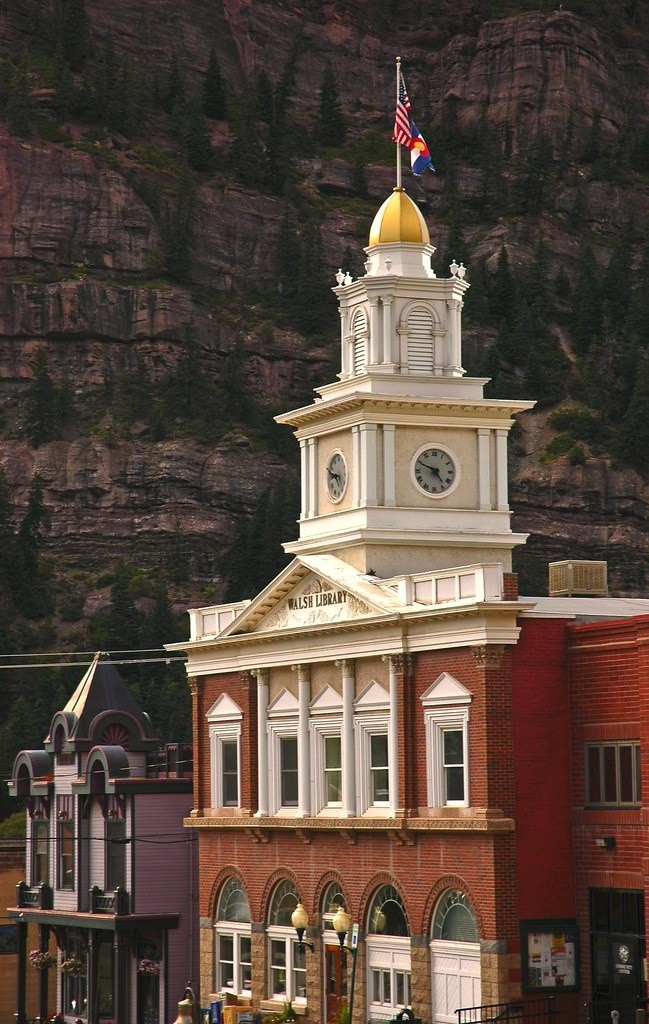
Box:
<box><xmin>325</xmin><ymin>449</ymin><xmax>350</xmax><ymax>504</ymax></box>
<box><xmin>411</xmin><ymin>442</ymin><xmax>461</xmax><ymax>500</ymax></box>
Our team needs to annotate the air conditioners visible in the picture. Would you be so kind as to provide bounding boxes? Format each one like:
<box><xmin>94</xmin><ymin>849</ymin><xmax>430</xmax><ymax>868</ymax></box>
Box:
<box><xmin>549</xmin><ymin>560</ymin><xmax>609</xmax><ymax>598</ymax></box>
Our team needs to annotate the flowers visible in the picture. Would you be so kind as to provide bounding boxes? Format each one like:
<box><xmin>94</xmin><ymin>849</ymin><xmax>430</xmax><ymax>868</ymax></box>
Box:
<box><xmin>59</xmin><ymin>955</ymin><xmax>86</xmax><ymax>975</ymax></box>
<box><xmin>29</xmin><ymin>948</ymin><xmax>57</xmax><ymax>975</ymax></box>
<box><xmin>137</xmin><ymin>959</ymin><xmax>161</xmax><ymax>976</ymax></box>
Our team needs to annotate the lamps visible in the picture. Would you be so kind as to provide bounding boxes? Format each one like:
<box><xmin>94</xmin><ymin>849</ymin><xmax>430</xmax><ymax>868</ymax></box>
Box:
<box><xmin>291</xmin><ymin>897</ymin><xmax>314</xmax><ymax>955</ymax></box>
<box><xmin>595</xmin><ymin>835</ymin><xmax>615</xmax><ymax>849</ymax></box>
<box><xmin>332</xmin><ymin>897</ymin><xmax>356</xmax><ymax>954</ymax></box>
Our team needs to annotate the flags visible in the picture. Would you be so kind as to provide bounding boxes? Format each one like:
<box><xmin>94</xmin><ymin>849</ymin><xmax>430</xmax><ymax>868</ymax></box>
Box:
<box><xmin>392</xmin><ymin>72</ymin><xmax>436</xmax><ymax>175</ymax></box>
